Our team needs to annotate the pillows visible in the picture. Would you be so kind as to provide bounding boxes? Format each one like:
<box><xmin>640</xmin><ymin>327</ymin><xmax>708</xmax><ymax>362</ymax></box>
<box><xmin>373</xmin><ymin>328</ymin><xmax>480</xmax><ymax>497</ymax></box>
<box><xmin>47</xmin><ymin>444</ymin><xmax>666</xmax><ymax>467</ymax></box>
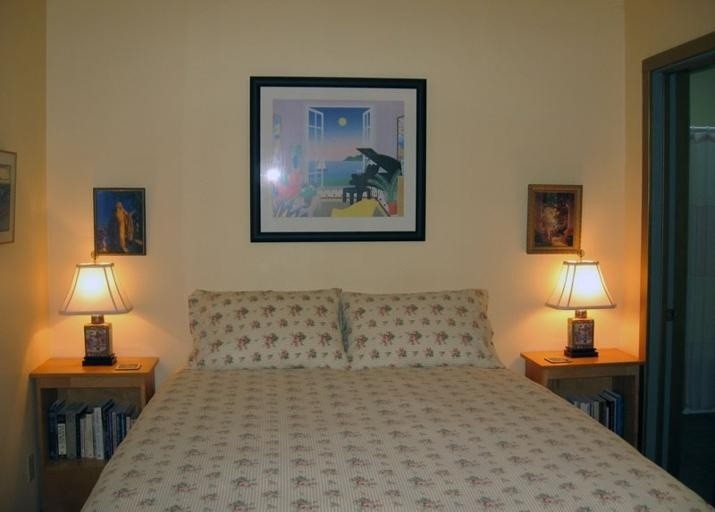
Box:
<box><xmin>188</xmin><ymin>288</ymin><xmax>348</xmax><ymax>369</ymax></box>
<box><xmin>343</xmin><ymin>289</ymin><xmax>505</xmax><ymax>367</ymax></box>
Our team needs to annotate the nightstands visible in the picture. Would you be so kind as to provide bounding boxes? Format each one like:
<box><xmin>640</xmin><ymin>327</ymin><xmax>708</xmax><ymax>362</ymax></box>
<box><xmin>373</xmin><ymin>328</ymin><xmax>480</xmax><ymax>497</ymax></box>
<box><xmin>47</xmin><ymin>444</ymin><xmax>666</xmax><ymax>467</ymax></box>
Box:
<box><xmin>521</xmin><ymin>349</ymin><xmax>640</xmax><ymax>446</ymax></box>
<box><xmin>30</xmin><ymin>357</ymin><xmax>161</xmax><ymax>511</ymax></box>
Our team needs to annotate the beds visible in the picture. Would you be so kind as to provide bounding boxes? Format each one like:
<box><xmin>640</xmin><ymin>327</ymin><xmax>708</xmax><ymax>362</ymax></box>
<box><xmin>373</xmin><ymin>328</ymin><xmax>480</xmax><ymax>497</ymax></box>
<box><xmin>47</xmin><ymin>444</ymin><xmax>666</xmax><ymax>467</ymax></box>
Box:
<box><xmin>79</xmin><ymin>364</ymin><xmax>715</xmax><ymax>511</ymax></box>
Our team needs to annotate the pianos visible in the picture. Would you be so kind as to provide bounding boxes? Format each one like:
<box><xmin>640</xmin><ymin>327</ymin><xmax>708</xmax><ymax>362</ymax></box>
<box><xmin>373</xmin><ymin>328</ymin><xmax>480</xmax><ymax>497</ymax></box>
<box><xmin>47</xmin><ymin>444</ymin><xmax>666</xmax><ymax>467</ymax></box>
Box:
<box><xmin>350</xmin><ymin>148</ymin><xmax>401</xmax><ymax>202</ymax></box>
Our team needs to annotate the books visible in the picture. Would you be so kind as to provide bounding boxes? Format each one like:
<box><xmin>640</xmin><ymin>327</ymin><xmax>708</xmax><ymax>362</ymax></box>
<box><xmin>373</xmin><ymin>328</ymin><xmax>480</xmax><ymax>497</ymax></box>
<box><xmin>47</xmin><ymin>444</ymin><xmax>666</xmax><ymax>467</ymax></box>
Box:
<box><xmin>46</xmin><ymin>398</ymin><xmax>139</xmax><ymax>462</ymax></box>
<box><xmin>565</xmin><ymin>388</ymin><xmax>624</xmax><ymax>438</ymax></box>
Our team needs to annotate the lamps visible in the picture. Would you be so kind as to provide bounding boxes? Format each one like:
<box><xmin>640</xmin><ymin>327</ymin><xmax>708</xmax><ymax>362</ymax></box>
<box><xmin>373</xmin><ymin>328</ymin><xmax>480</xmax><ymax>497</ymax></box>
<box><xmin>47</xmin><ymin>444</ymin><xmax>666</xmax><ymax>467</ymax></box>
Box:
<box><xmin>60</xmin><ymin>262</ymin><xmax>134</xmax><ymax>365</ymax></box>
<box><xmin>544</xmin><ymin>261</ymin><xmax>616</xmax><ymax>356</ymax></box>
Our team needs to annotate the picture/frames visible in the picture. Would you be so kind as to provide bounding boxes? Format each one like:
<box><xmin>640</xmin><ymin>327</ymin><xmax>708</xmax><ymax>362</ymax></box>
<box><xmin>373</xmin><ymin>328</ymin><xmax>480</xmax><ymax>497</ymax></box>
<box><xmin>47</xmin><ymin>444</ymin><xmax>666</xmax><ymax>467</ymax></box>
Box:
<box><xmin>528</xmin><ymin>184</ymin><xmax>583</xmax><ymax>254</ymax></box>
<box><xmin>249</xmin><ymin>77</ymin><xmax>427</xmax><ymax>243</ymax></box>
<box><xmin>0</xmin><ymin>149</ymin><xmax>17</xmax><ymax>243</ymax></box>
<box><xmin>93</xmin><ymin>188</ymin><xmax>146</xmax><ymax>256</ymax></box>
